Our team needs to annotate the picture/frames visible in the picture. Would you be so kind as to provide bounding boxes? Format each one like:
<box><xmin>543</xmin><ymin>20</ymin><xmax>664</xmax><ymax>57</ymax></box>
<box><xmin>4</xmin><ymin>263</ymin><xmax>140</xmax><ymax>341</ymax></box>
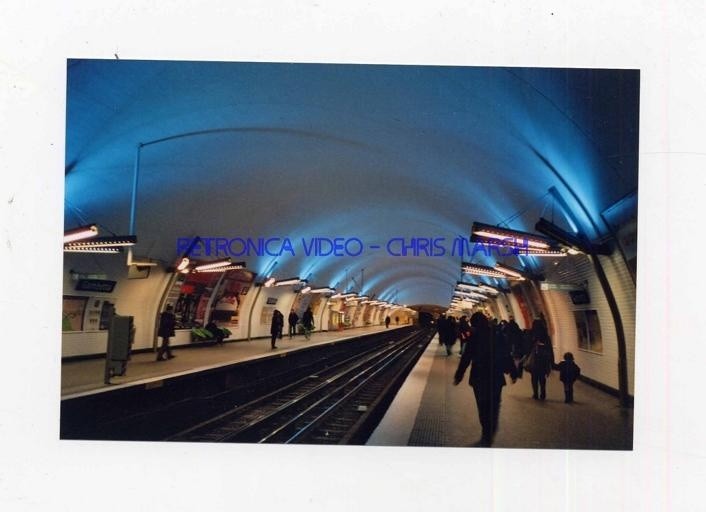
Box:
<box><xmin>64</xmin><ymin>212</ymin><xmax>613</xmax><ymax>312</ymax></box>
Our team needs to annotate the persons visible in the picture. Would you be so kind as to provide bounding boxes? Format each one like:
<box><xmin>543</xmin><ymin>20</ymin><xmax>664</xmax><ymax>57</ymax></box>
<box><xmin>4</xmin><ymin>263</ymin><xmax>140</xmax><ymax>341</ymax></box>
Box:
<box><xmin>385</xmin><ymin>315</ymin><xmax>390</xmax><ymax>328</ymax></box>
<box><xmin>454</xmin><ymin>311</ymin><xmax>519</xmax><ymax>447</ymax></box>
<box><xmin>435</xmin><ymin>310</ymin><xmax>549</xmax><ymax>380</ymax></box>
<box><xmin>303</xmin><ymin>307</ymin><xmax>315</xmax><ymax>340</ymax></box>
<box><xmin>154</xmin><ymin>304</ymin><xmax>177</xmax><ymax>361</ymax></box>
<box><xmin>271</xmin><ymin>309</ymin><xmax>280</xmax><ymax>350</ymax></box>
<box><xmin>520</xmin><ymin>319</ymin><xmax>555</xmax><ymax>400</ymax></box>
<box><xmin>278</xmin><ymin>311</ymin><xmax>285</xmax><ymax>338</ymax></box>
<box><xmin>549</xmin><ymin>351</ymin><xmax>581</xmax><ymax>403</ymax></box>
<box><xmin>205</xmin><ymin>318</ymin><xmax>224</xmax><ymax>347</ymax></box>
<box><xmin>288</xmin><ymin>308</ymin><xmax>299</xmax><ymax>337</ymax></box>
